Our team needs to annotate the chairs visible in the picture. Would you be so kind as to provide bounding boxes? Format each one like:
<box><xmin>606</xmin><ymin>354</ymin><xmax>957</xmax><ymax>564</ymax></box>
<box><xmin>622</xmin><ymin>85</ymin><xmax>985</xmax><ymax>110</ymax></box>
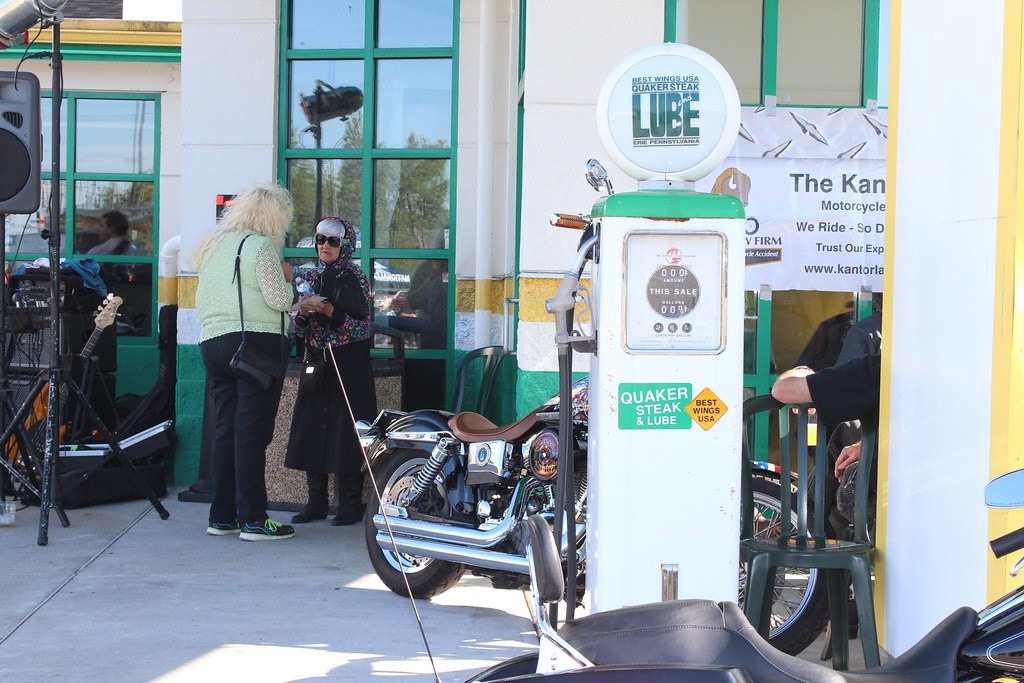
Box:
<box><xmin>452</xmin><ymin>344</ymin><xmax>506</xmax><ymax>416</ymax></box>
<box><xmin>741</xmin><ymin>394</ymin><xmax>879</xmax><ymax>669</ymax></box>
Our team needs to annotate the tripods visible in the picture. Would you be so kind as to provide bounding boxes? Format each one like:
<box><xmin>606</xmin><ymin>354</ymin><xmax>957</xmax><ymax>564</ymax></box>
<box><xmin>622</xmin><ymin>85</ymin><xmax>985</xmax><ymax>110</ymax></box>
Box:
<box><xmin>0</xmin><ymin>19</ymin><xmax>171</xmax><ymax>546</ymax></box>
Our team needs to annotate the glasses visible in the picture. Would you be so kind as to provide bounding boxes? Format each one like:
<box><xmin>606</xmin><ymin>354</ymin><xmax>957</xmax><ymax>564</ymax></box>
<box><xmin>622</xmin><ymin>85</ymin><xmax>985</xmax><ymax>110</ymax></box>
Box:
<box><xmin>315</xmin><ymin>232</ymin><xmax>343</xmax><ymax>247</ymax></box>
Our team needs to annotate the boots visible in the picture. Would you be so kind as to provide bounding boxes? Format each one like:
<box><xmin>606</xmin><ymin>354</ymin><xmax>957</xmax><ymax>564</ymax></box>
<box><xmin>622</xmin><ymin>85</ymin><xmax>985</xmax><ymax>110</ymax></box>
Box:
<box><xmin>330</xmin><ymin>471</ymin><xmax>362</xmax><ymax>526</ymax></box>
<box><xmin>291</xmin><ymin>470</ymin><xmax>330</xmax><ymax>523</ymax></box>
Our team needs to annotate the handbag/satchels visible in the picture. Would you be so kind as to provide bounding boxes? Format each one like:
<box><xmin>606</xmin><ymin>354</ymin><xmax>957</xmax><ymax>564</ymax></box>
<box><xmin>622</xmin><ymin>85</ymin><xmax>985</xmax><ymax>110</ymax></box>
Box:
<box><xmin>97</xmin><ymin>239</ymin><xmax>140</xmax><ymax>313</ymax></box>
<box><xmin>229</xmin><ymin>342</ymin><xmax>286</xmax><ymax>391</ymax></box>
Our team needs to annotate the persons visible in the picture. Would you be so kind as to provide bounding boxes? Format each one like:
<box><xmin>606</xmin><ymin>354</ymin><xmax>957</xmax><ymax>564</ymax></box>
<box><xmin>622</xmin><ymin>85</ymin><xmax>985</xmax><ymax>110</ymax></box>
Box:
<box><xmin>176</xmin><ymin>182</ymin><xmax>295</xmax><ymax>540</ymax></box>
<box><xmin>283</xmin><ymin>217</ymin><xmax>380</xmax><ymax>526</ymax></box>
<box><xmin>83</xmin><ymin>211</ymin><xmax>145</xmax><ymax>277</ymax></box>
<box><xmin>404</xmin><ymin>227</ymin><xmax>450</xmax><ymax>322</ymax></box>
<box><xmin>772</xmin><ymin>291</ymin><xmax>883</xmax><ymax>530</ymax></box>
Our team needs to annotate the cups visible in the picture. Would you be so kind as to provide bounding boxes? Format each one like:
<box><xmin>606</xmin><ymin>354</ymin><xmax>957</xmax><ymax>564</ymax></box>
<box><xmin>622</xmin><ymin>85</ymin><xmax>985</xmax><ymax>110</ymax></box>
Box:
<box><xmin>0</xmin><ymin>501</ymin><xmax>16</xmax><ymax>527</ymax></box>
<box><xmin>807</xmin><ymin>408</ymin><xmax>818</xmax><ymax>447</ymax></box>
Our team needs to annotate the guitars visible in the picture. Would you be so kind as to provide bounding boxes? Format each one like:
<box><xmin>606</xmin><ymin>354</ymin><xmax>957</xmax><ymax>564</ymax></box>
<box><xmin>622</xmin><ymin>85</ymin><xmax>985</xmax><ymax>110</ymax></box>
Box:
<box><xmin>4</xmin><ymin>291</ymin><xmax>124</xmax><ymax>469</ymax></box>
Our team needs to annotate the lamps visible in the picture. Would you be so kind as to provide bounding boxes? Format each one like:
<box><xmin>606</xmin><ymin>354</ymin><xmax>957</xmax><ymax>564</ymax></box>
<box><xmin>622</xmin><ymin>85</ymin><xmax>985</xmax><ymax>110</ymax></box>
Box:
<box><xmin>0</xmin><ymin>0</ymin><xmax>172</xmax><ymax>549</ymax></box>
<box><xmin>298</xmin><ymin>77</ymin><xmax>364</xmax><ymax>250</ymax></box>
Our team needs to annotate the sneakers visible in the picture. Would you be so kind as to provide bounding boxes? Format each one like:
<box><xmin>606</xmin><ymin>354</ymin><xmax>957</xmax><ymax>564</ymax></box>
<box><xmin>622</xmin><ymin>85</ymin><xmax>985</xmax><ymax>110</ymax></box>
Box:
<box><xmin>240</xmin><ymin>519</ymin><xmax>296</xmax><ymax>540</ymax></box>
<box><xmin>208</xmin><ymin>518</ymin><xmax>241</xmax><ymax>535</ymax></box>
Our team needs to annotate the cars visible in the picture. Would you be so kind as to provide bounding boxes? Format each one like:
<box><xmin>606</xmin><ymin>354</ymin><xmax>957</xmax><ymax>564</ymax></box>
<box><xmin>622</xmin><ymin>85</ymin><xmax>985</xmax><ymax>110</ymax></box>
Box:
<box><xmin>389</xmin><ymin>288</ymin><xmax>415</xmax><ymax>315</ymax></box>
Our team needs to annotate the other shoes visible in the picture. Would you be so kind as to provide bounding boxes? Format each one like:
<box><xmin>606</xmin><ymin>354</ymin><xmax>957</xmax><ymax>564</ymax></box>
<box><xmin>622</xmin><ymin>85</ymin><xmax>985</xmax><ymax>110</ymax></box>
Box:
<box><xmin>844</xmin><ymin>597</ymin><xmax>860</xmax><ymax>639</ymax></box>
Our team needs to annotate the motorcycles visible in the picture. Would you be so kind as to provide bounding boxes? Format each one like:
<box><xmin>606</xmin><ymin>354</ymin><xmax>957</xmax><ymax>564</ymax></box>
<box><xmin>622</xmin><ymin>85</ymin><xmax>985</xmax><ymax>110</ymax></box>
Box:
<box><xmin>461</xmin><ymin>468</ymin><xmax>1024</xmax><ymax>683</ymax></box>
<box><xmin>352</xmin><ymin>155</ymin><xmax>836</xmax><ymax>654</ymax></box>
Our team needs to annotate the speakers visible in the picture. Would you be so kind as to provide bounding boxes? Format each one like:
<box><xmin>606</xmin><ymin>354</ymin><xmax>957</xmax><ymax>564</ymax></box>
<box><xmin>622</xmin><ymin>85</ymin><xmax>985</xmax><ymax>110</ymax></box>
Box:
<box><xmin>0</xmin><ymin>71</ymin><xmax>43</xmax><ymax>215</ymax></box>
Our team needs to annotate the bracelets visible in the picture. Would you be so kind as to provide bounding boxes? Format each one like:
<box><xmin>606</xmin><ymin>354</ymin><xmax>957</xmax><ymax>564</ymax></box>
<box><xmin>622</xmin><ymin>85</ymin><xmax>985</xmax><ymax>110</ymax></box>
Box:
<box><xmin>327</xmin><ymin>306</ymin><xmax>334</xmax><ymax>313</ymax></box>
<box><xmin>793</xmin><ymin>365</ymin><xmax>812</xmax><ymax>371</ymax></box>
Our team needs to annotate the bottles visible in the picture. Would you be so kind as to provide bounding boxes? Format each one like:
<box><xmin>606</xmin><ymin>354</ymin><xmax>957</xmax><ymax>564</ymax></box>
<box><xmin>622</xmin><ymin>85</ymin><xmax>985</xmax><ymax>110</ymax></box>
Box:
<box><xmin>295</xmin><ymin>277</ymin><xmax>317</xmax><ymax>313</ymax></box>
